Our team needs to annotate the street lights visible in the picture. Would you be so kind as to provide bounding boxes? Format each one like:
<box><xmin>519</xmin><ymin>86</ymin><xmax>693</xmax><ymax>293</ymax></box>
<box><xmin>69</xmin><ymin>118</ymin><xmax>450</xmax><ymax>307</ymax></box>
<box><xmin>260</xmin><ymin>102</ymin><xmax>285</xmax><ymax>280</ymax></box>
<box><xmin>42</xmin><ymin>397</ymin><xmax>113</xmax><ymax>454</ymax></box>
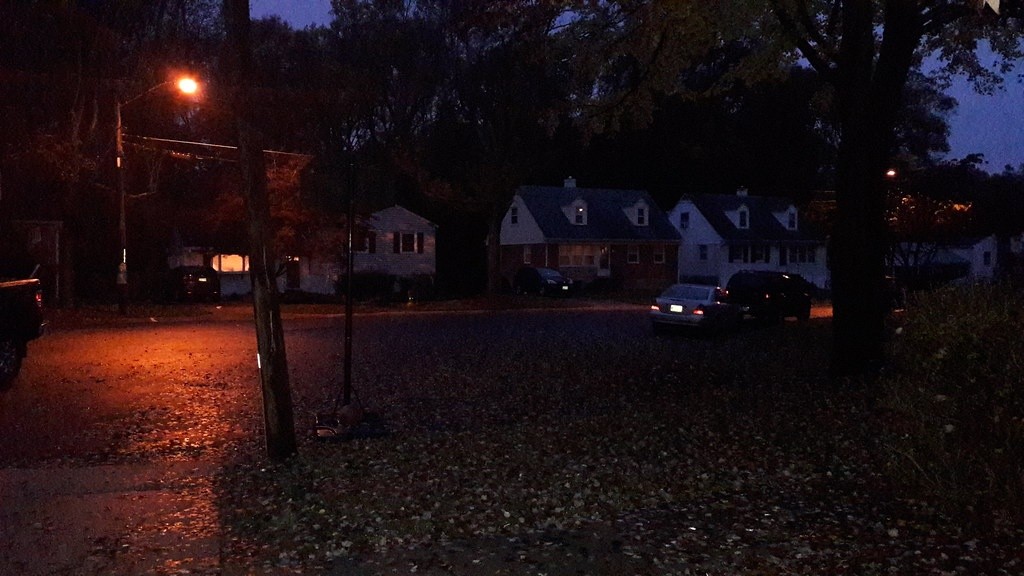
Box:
<box><xmin>116</xmin><ymin>76</ymin><xmax>203</xmax><ymax>308</ymax></box>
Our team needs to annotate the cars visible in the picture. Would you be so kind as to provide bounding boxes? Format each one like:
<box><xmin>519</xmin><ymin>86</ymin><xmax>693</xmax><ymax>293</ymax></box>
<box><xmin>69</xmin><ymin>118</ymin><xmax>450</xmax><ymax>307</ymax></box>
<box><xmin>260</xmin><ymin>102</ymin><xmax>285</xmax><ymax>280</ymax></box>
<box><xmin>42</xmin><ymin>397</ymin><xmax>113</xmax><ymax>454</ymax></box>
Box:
<box><xmin>165</xmin><ymin>265</ymin><xmax>220</xmax><ymax>302</ymax></box>
<box><xmin>514</xmin><ymin>267</ymin><xmax>575</xmax><ymax>300</ymax></box>
<box><xmin>648</xmin><ymin>283</ymin><xmax>743</xmax><ymax>339</ymax></box>
<box><xmin>0</xmin><ymin>276</ymin><xmax>49</xmax><ymax>394</ymax></box>
<box><xmin>726</xmin><ymin>270</ymin><xmax>813</xmax><ymax>320</ymax></box>
